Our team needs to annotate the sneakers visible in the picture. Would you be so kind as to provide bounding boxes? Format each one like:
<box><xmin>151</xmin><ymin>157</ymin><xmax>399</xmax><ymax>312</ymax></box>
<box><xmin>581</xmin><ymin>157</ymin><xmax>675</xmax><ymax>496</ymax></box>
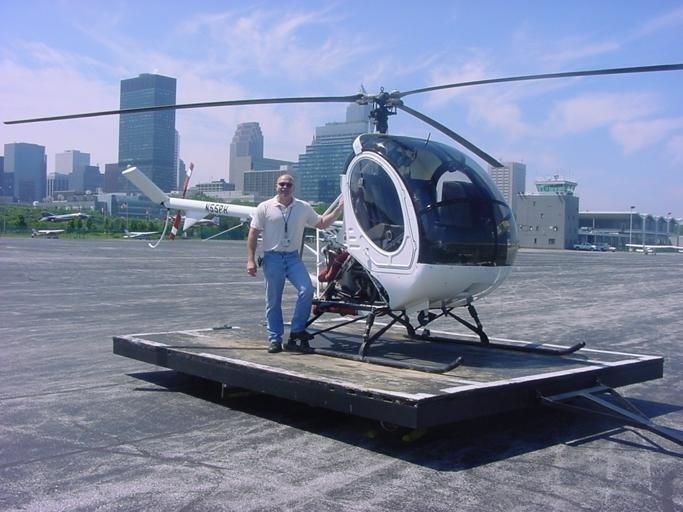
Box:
<box><xmin>268</xmin><ymin>343</ymin><xmax>283</xmax><ymax>352</ymax></box>
<box><xmin>290</xmin><ymin>330</ymin><xmax>314</xmax><ymax>340</ymax></box>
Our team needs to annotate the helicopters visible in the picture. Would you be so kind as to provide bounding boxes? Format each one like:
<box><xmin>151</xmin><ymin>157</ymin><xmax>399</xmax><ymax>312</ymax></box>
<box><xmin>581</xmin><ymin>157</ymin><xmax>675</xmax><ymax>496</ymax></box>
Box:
<box><xmin>2</xmin><ymin>62</ymin><xmax>682</xmax><ymax>373</ymax></box>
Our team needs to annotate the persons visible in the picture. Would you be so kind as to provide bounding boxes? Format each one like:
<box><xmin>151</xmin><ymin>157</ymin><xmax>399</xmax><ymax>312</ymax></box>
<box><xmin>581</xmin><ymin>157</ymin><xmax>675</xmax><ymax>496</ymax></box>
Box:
<box><xmin>246</xmin><ymin>173</ymin><xmax>344</xmax><ymax>353</ymax></box>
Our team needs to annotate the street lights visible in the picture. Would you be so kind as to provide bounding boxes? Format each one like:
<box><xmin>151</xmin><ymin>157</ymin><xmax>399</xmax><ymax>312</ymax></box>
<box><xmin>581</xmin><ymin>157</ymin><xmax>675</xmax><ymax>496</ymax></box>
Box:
<box><xmin>667</xmin><ymin>212</ymin><xmax>672</xmax><ymax>245</ymax></box>
<box><xmin>630</xmin><ymin>205</ymin><xmax>636</xmax><ymax>243</ymax></box>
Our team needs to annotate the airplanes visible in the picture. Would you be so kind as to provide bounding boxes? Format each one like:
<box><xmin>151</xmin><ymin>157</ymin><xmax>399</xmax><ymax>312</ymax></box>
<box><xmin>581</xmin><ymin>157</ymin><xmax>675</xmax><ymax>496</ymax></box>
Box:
<box><xmin>38</xmin><ymin>211</ymin><xmax>88</xmax><ymax>223</ymax></box>
<box><xmin>31</xmin><ymin>228</ymin><xmax>65</xmax><ymax>239</ymax></box>
<box><xmin>163</xmin><ymin>215</ymin><xmax>220</xmax><ymax>226</ymax></box>
<box><xmin>123</xmin><ymin>229</ymin><xmax>160</xmax><ymax>239</ymax></box>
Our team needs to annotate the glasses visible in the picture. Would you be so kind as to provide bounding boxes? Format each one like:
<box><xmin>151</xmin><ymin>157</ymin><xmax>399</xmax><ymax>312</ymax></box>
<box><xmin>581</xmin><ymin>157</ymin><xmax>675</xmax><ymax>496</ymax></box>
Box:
<box><xmin>279</xmin><ymin>182</ymin><xmax>292</xmax><ymax>187</ymax></box>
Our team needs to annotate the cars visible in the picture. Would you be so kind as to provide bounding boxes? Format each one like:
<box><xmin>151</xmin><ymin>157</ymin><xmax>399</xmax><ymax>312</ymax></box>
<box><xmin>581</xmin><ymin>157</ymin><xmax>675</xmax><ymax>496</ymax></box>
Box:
<box><xmin>573</xmin><ymin>241</ymin><xmax>616</xmax><ymax>252</ymax></box>
